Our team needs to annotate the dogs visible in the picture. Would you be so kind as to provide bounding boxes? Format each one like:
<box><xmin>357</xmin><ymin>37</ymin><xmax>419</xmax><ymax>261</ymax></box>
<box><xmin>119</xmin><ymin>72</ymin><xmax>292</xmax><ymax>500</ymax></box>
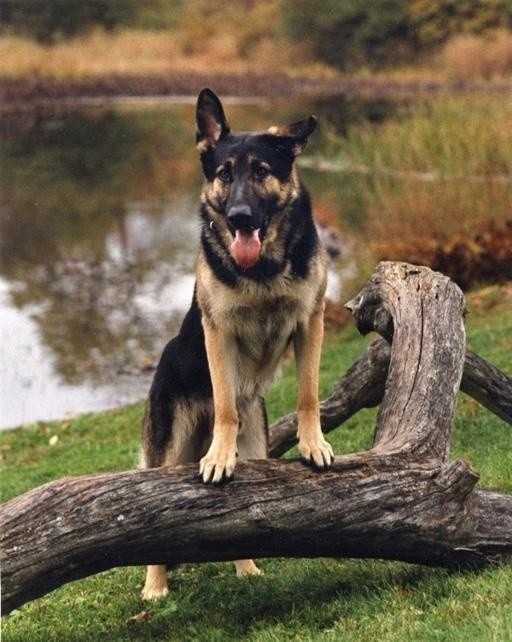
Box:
<box><xmin>138</xmin><ymin>86</ymin><xmax>335</xmax><ymax>602</ymax></box>
